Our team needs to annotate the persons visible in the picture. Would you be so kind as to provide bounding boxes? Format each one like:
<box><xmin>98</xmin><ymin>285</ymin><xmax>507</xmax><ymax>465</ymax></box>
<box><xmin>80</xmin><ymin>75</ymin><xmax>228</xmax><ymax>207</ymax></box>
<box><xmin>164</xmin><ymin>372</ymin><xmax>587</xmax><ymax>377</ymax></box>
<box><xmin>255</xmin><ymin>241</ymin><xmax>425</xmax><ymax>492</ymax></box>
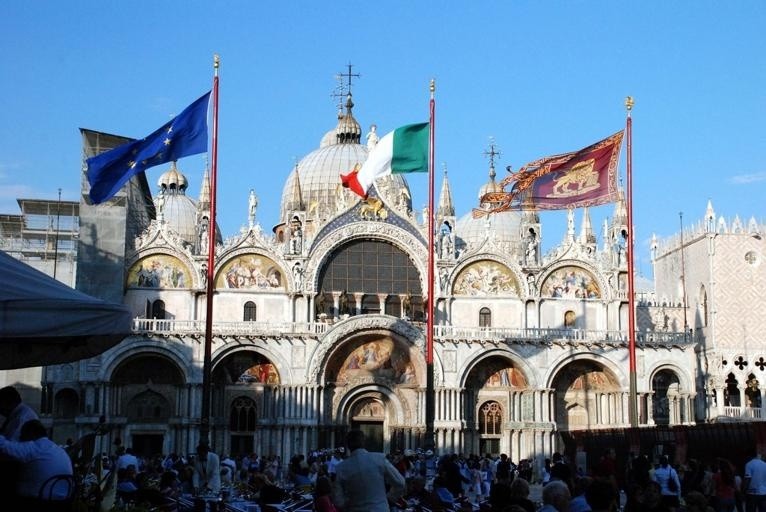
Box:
<box><xmin>232</xmin><ymin>357</ymin><xmax>271</xmax><ymax>383</ymax></box>
<box><xmin>225</xmin><ymin>260</ymin><xmax>278</xmax><ymax>288</ymax></box>
<box><xmin>476</xmin><ymin>362</ymin><xmax>515</xmax><ymax>388</ymax></box>
<box><xmin>135</xmin><ymin>260</ymin><xmax>186</xmax><ymax>288</ymax></box>
<box><xmin>63</xmin><ymin>428</ymin><xmax>766</xmax><ymax>512</ymax></box>
<box><xmin>346</xmin><ymin>342</ymin><xmax>391</xmax><ymax>370</ymax></box>
<box><xmin>0</xmin><ymin>386</ymin><xmax>72</xmax><ymax>512</ymax></box>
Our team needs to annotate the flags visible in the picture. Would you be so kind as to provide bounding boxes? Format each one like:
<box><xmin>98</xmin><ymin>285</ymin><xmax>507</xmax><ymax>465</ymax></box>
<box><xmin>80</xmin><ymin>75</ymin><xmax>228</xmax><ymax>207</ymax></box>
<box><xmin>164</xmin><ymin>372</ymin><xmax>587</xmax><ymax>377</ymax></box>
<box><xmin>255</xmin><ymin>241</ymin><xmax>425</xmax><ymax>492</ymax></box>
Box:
<box><xmin>339</xmin><ymin>120</ymin><xmax>430</xmax><ymax>200</ymax></box>
<box><xmin>85</xmin><ymin>90</ymin><xmax>211</xmax><ymax>206</ymax></box>
<box><xmin>472</xmin><ymin>131</ymin><xmax>623</xmax><ymax>219</ymax></box>
